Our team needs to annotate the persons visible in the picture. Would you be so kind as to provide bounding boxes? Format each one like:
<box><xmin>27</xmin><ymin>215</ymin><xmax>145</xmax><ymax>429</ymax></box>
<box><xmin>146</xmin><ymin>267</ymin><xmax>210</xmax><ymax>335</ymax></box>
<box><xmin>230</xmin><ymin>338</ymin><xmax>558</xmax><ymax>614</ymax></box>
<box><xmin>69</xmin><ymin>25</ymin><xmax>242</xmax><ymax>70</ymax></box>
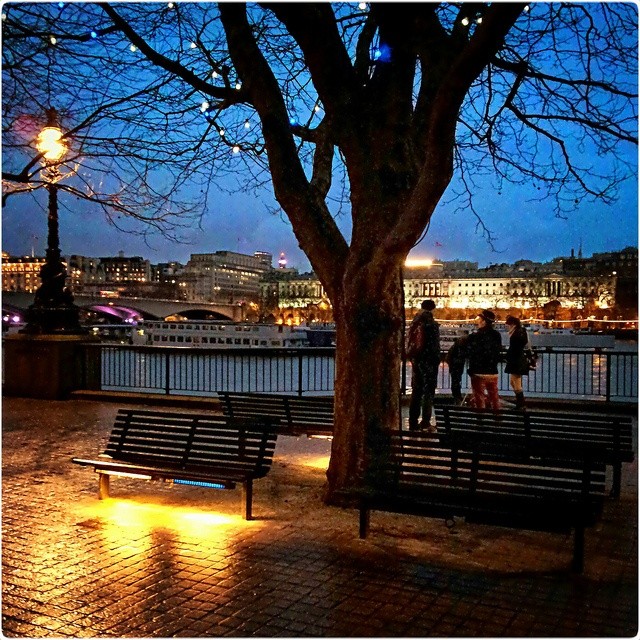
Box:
<box><xmin>466</xmin><ymin>309</ymin><xmax>502</xmax><ymax>420</ymax></box>
<box><xmin>408</xmin><ymin>299</ymin><xmax>440</xmax><ymax>432</ymax></box>
<box><xmin>504</xmin><ymin>315</ymin><xmax>538</xmax><ymax>411</ymax></box>
<box><xmin>446</xmin><ymin>334</ymin><xmax>470</xmax><ymax>407</ymax></box>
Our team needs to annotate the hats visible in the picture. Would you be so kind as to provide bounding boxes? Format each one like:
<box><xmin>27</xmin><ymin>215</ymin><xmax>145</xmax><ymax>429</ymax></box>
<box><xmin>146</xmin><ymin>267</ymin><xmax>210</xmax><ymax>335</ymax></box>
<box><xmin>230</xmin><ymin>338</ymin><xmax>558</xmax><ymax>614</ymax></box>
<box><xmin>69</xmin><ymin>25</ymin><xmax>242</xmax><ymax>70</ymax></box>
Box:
<box><xmin>421</xmin><ymin>299</ymin><xmax>435</xmax><ymax>311</ymax></box>
<box><xmin>477</xmin><ymin>310</ymin><xmax>495</xmax><ymax>322</ymax></box>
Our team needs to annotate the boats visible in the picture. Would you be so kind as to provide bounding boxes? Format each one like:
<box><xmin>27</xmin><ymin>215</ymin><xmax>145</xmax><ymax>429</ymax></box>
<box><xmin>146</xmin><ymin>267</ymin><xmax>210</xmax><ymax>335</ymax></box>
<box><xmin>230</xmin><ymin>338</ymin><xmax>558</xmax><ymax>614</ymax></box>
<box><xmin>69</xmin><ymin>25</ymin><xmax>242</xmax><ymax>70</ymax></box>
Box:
<box><xmin>402</xmin><ymin>324</ymin><xmax>464</xmax><ymax>354</ymax></box>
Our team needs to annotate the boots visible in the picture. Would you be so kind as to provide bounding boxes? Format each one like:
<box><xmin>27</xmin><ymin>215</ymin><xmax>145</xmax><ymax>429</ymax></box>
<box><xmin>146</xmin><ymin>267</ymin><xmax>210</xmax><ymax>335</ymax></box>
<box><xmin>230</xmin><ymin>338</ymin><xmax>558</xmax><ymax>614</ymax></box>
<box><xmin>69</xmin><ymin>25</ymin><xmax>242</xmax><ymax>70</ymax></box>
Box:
<box><xmin>512</xmin><ymin>389</ymin><xmax>527</xmax><ymax>411</ymax></box>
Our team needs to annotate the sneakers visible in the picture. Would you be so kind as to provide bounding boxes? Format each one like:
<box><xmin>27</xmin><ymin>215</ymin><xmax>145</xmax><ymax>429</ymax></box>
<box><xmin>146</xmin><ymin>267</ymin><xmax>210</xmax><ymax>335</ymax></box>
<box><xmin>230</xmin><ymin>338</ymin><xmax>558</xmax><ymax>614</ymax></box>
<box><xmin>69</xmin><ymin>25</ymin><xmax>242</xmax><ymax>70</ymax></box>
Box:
<box><xmin>420</xmin><ymin>424</ymin><xmax>437</xmax><ymax>434</ymax></box>
<box><xmin>409</xmin><ymin>427</ymin><xmax>427</xmax><ymax>433</ymax></box>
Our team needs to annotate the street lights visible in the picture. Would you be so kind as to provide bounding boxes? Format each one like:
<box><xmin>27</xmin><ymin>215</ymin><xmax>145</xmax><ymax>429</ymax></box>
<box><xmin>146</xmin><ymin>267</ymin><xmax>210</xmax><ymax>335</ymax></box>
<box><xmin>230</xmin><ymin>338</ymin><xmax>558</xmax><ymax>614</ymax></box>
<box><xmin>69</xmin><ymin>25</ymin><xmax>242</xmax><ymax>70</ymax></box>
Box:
<box><xmin>17</xmin><ymin>106</ymin><xmax>91</xmax><ymax>337</ymax></box>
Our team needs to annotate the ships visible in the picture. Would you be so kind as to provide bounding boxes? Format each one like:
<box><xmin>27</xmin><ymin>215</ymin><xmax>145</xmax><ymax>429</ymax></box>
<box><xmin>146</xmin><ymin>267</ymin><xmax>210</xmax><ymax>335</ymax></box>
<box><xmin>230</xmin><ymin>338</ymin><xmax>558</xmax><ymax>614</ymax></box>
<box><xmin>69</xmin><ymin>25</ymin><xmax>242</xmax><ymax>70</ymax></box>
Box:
<box><xmin>112</xmin><ymin>317</ymin><xmax>336</xmax><ymax>358</ymax></box>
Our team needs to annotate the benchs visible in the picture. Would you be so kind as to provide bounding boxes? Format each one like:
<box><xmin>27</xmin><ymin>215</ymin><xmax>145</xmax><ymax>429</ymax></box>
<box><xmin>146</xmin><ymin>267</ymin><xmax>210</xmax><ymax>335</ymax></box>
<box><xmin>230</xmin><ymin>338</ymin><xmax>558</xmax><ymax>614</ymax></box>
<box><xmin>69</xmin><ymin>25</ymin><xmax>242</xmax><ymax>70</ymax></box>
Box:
<box><xmin>434</xmin><ymin>402</ymin><xmax>634</xmax><ymax>497</ymax></box>
<box><xmin>323</xmin><ymin>427</ymin><xmax>606</xmax><ymax>574</ymax></box>
<box><xmin>71</xmin><ymin>409</ymin><xmax>281</xmax><ymax>519</ymax></box>
<box><xmin>218</xmin><ymin>390</ymin><xmax>334</xmax><ymax>456</ymax></box>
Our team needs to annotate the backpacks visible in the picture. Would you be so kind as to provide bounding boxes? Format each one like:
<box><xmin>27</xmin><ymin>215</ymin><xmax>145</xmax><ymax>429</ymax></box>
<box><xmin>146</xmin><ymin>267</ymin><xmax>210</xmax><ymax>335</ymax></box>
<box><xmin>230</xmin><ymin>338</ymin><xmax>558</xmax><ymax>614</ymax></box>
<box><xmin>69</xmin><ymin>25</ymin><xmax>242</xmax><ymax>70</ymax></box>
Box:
<box><xmin>405</xmin><ymin>319</ymin><xmax>425</xmax><ymax>359</ymax></box>
